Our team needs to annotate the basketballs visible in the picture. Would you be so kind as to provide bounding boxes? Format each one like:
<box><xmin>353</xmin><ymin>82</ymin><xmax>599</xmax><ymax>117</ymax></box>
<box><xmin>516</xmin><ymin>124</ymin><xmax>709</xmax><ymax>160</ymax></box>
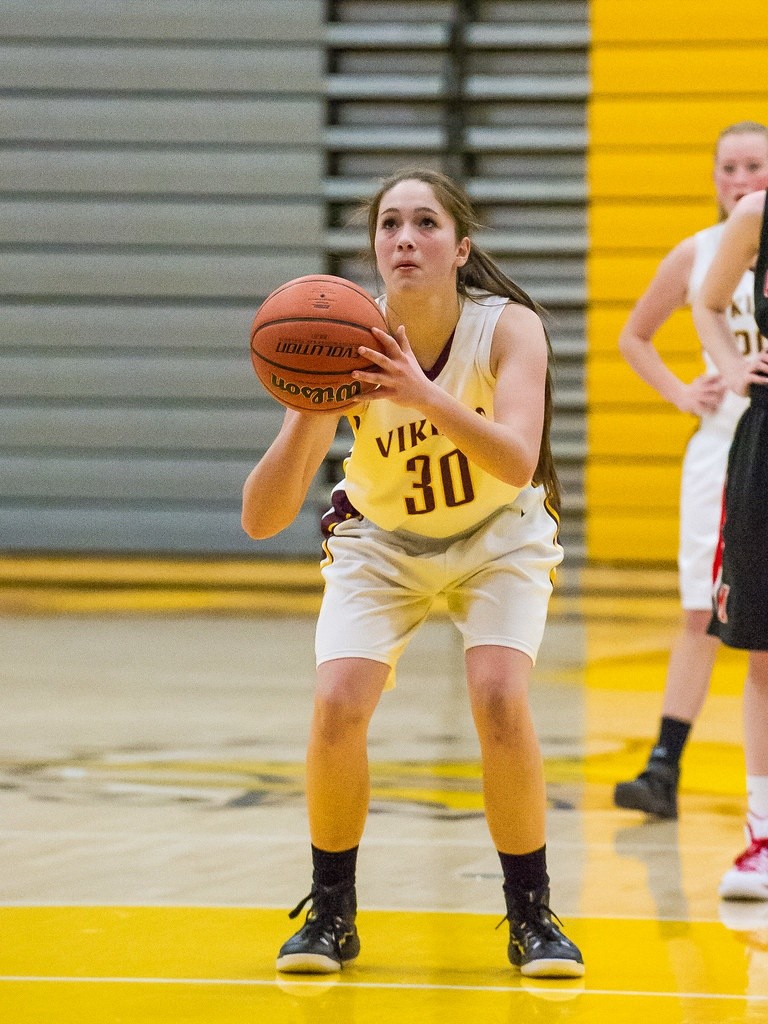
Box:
<box><xmin>249</xmin><ymin>273</ymin><xmax>392</xmax><ymax>417</ymax></box>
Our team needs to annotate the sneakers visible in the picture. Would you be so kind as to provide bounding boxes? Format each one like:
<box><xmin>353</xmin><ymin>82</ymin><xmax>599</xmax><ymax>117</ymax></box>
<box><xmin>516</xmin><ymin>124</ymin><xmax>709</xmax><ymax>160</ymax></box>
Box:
<box><xmin>276</xmin><ymin>876</ymin><xmax>360</xmax><ymax>974</ymax></box>
<box><xmin>504</xmin><ymin>885</ymin><xmax>585</xmax><ymax>978</ymax></box>
<box><xmin>615</xmin><ymin>746</ymin><xmax>680</xmax><ymax>816</ymax></box>
<box><xmin>718</xmin><ymin>824</ymin><xmax>768</xmax><ymax>900</ymax></box>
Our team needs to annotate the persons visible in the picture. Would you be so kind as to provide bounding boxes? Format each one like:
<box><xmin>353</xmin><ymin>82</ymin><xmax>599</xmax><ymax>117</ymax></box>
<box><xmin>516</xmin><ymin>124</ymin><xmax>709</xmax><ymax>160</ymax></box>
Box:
<box><xmin>235</xmin><ymin>169</ymin><xmax>589</xmax><ymax>979</ymax></box>
<box><xmin>610</xmin><ymin>118</ymin><xmax>768</xmax><ymax>904</ymax></box>
